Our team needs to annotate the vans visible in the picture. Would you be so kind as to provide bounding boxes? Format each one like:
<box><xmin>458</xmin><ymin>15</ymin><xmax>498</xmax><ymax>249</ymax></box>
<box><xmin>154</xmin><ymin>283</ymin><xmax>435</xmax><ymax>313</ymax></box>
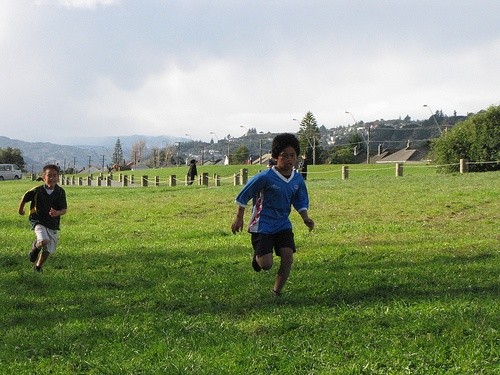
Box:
<box><xmin>0</xmin><ymin>164</ymin><xmax>22</xmax><ymax>181</ymax></box>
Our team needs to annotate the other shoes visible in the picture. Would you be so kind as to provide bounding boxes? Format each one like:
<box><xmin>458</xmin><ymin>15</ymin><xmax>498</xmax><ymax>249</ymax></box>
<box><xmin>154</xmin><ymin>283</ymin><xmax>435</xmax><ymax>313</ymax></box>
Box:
<box><xmin>272</xmin><ymin>289</ymin><xmax>280</xmax><ymax>296</ymax></box>
<box><xmin>252</xmin><ymin>251</ymin><xmax>261</xmax><ymax>272</ymax></box>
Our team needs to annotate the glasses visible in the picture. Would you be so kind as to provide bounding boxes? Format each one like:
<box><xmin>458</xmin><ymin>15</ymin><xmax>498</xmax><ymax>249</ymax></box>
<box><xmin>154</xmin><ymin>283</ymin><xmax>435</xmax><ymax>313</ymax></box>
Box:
<box><xmin>278</xmin><ymin>152</ymin><xmax>297</xmax><ymax>159</ymax></box>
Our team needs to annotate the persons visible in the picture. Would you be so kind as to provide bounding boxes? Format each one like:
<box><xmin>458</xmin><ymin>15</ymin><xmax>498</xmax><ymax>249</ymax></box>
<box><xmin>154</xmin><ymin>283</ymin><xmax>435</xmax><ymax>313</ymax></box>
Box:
<box><xmin>265</xmin><ymin>155</ymin><xmax>307</xmax><ymax>180</ymax></box>
<box><xmin>230</xmin><ymin>133</ymin><xmax>314</xmax><ymax>298</ymax></box>
<box><xmin>18</xmin><ymin>165</ymin><xmax>68</xmax><ymax>272</ymax></box>
<box><xmin>187</xmin><ymin>160</ymin><xmax>197</xmax><ymax>184</ymax></box>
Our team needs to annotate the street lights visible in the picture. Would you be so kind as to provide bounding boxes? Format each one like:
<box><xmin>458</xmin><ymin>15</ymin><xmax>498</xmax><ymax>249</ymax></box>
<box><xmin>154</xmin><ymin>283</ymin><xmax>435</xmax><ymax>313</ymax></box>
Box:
<box><xmin>210</xmin><ymin>133</ymin><xmax>229</xmax><ymax>165</ymax></box>
<box><xmin>423</xmin><ymin>105</ymin><xmax>443</xmax><ymax>134</ymax></box>
<box><xmin>240</xmin><ymin>125</ymin><xmax>262</xmax><ymax>164</ymax></box>
<box><xmin>163</xmin><ymin>140</ymin><xmax>180</xmax><ymax>167</ymax></box>
<box><xmin>346</xmin><ymin>112</ymin><xmax>370</xmax><ymax>164</ymax></box>
<box><xmin>186</xmin><ymin>134</ymin><xmax>204</xmax><ymax>166</ymax></box>
<box><xmin>294</xmin><ymin>118</ymin><xmax>315</xmax><ymax>164</ymax></box>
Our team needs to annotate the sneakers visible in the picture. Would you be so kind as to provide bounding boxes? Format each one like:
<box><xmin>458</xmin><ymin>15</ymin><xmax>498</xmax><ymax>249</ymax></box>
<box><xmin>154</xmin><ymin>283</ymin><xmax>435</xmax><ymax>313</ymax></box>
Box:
<box><xmin>29</xmin><ymin>241</ymin><xmax>41</xmax><ymax>263</ymax></box>
<box><xmin>35</xmin><ymin>265</ymin><xmax>43</xmax><ymax>273</ymax></box>
